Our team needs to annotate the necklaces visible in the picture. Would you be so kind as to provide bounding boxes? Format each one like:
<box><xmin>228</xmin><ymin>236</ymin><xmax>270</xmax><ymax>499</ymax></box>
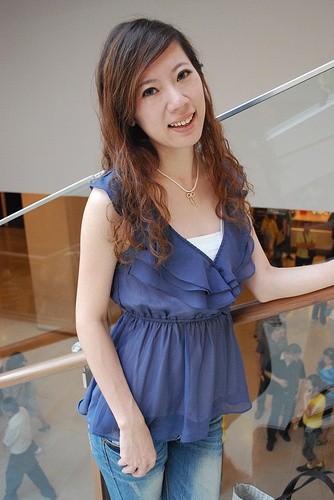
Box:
<box><xmin>144</xmin><ymin>154</ymin><xmax>199</xmax><ymax>206</ymax></box>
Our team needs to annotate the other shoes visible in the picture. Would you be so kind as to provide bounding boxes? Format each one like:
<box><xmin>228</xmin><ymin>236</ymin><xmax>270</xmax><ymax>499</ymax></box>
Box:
<box><xmin>4</xmin><ymin>495</ymin><xmax>18</xmax><ymax>500</ymax></box>
<box><xmin>34</xmin><ymin>448</ymin><xmax>42</xmax><ymax>454</ymax></box>
<box><xmin>320</xmin><ymin>317</ymin><xmax>326</xmax><ymax>325</ymax></box>
<box><xmin>312</xmin><ymin>316</ymin><xmax>318</xmax><ymax>321</ymax></box>
<box><xmin>297</xmin><ymin>461</ymin><xmax>324</xmax><ymax>472</ymax></box>
<box><xmin>267</xmin><ymin>436</ymin><xmax>276</xmax><ymax>451</ymax></box>
<box><xmin>41</xmin><ymin>489</ymin><xmax>56</xmax><ymax>499</ymax></box>
<box><xmin>38</xmin><ymin>425</ymin><xmax>51</xmax><ymax>432</ymax></box>
<box><xmin>316</xmin><ymin>439</ymin><xmax>327</xmax><ymax>446</ymax></box>
<box><xmin>254</xmin><ymin>408</ymin><xmax>265</xmax><ymax>418</ymax></box>
<box><xmin>280</xmin><ymin>431</ymin><xmax>290</xmax><ymax>441</ymax></box>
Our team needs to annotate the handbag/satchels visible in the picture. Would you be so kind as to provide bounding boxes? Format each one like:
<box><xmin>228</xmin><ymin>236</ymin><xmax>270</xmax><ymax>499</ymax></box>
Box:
<box><xmin>233</xmin><ymin>468</ymin><xmax>334</xmax><ymax>500</ymax></box>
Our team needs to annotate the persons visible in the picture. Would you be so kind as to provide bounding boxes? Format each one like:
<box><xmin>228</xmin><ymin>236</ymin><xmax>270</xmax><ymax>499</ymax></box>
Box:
<box><xmin>75</xmin><ymin>18</ymin><xmax>334</xmax><ymax>500</ymax></box>
<box><xmin>252</xmin><ymin>207</ymin><xmax>334</xmax><ymax>472</ymax></box>
<box><xmin>0</xmin><ymin>351</ymin><xmax>58</xmax><ymax>500</ymax></box>
